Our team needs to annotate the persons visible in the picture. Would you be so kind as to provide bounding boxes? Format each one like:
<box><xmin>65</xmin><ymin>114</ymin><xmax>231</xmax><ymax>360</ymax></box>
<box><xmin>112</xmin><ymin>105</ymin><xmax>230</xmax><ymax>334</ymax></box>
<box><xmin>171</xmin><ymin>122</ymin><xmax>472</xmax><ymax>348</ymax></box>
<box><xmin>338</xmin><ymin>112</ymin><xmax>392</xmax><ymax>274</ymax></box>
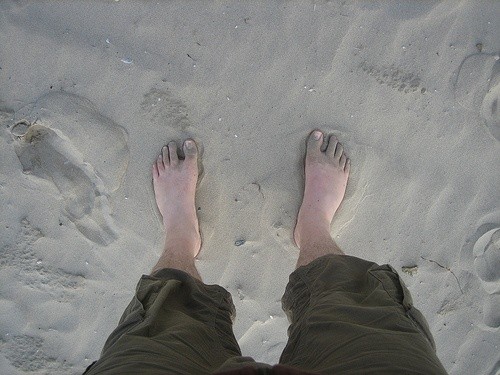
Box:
<box><xmin>83</xmin><ymin>129</ymin><xmax>450</xmax><ymax>375</ymax></box>
